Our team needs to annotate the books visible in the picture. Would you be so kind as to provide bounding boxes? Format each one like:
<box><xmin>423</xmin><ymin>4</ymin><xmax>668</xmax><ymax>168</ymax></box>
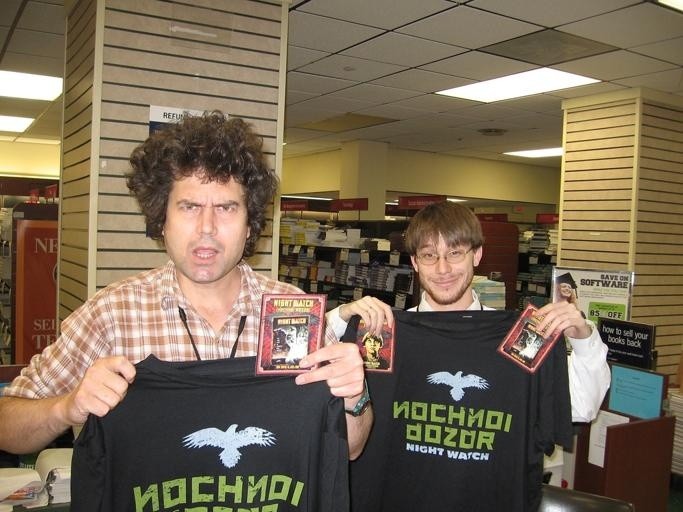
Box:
<box><xmin>0</xmin><ymin>447</ymin><xmax>75</xmax><ymax>512</ymax></box>
<box><xmin>660</xmin><ymin>385</ymin><xmax>682</xmax><ymax>475</ymax></box>
<box><xmin>496</xmin><ymin>303</ymin><xmax>562</xmax><ymax>375</ymax></box>
<box><xmin>251</xmin><ymin>292</ymin><xmax>327</xmax><ymax>376</ymax></box>
<box><xmin>355</xmin><ymin>315</ymin><xmax>396</xmax><ymax>372</ymax></box>
<box><xmin>277</xmin><ymin>214</ymin><xmax>558</xmax><ymax>317</ymax></box>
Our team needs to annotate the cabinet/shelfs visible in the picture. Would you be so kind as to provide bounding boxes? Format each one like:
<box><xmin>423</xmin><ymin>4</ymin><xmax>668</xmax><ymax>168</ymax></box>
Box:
<box><xmin>515</xmin><ymin>217</ymin><xmax>558</xmax><ymax>317</ymax></box>
<box><xmin>276</xmin><ymin>214</ymin><xmax>516</xmax><ymax>328</ymax></box>
<box><xmin>541</xmin><ymin>372</ymin><xmax>677</xmax><ymax>512</ymax></box>
<box><xmin>0</xmin><ymin>199</ymin><xmax>59</xmax><ymax>366</ymax></box>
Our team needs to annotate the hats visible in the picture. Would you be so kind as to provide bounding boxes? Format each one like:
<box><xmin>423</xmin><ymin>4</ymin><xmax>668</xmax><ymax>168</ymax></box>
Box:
<box><xmin>555</xmin><ymin>273</ymin><xmax>578</xmax><ymax>298</ymax></box>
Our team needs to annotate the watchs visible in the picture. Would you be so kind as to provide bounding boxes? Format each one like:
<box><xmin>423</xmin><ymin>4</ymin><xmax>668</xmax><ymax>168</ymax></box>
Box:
<box><xmin>342</xmin><ymin>379</ymin><xmax>373</xmax><ymax>419</ymax></box>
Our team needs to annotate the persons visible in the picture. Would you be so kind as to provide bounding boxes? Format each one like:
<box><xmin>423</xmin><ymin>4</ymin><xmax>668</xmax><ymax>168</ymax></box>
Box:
<box><xmin>271</xmin><ymin>324</ymin><xmax>306</xmax><ymax>364</ymax></box>
<box><xmin>360</xmin><ymin>331</ymin><xmax>388</xmax><ymax>370</ymax></box>
<box><xmin>326</xmin><ymin>201</ymin><xmax>613</xmax><ymax>427</ymax></box>
<box><xmin>1</xmin><ymin>110</ymin><xmax>375</xmax><ymax>466</ymax></box>
<box><xmin>553</xmin><ymin>271</ymin><xmax>586</xmax><ymax>323</ymax></box>
<box><xmin>519</xmin><ymin>327</ymin><xmax>539</xmax><ymax>359</ymax></box>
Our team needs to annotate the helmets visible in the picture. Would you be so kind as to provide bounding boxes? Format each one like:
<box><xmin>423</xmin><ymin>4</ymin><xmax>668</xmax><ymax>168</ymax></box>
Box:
<box><xmin>362</xmin><ymin>331</ymin><xmax>384</xmax><ymax>348</ymax></box>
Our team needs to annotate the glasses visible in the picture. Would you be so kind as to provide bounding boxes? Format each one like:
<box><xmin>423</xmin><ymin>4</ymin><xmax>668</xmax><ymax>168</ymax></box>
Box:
<box><xmin>417</xmin><ymin>247</ymin><xmax>473</xmax><ymax>266</ymax></box>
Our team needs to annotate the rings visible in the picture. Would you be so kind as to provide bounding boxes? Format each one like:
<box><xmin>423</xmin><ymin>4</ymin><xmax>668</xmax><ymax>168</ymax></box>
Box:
<box><xmin>366</xmin><ymin>306</ymin><xmax>374</xmax><ymax>314</ymax></box>
